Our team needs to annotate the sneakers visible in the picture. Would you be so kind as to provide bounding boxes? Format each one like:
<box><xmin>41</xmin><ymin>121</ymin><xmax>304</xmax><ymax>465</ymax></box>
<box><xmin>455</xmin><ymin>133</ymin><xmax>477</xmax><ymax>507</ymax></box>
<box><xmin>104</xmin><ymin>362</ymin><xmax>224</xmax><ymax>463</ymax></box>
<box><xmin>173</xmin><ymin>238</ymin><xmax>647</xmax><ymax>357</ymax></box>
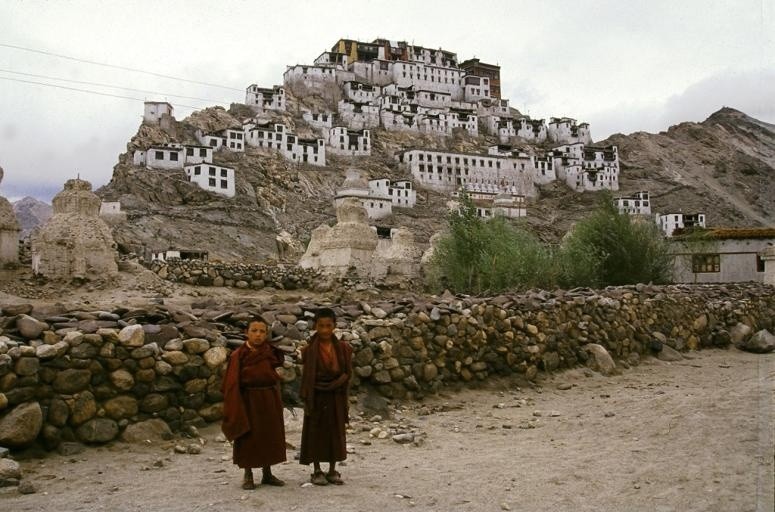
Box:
<box><xmin>243</xmin><ymin>479</ymin><xmax>254</xmax><ymax>490</ymax></box>
<box><xmin>311</xmin><ymin>471</ymin><xmax>329</xmax><ymax>485</ymax></box>
<box><xmin>262</xmin><ymin>475</ymin><xmax>284</xmax><ymax>486</ymax></box>
<box><xmin>326</xmin><ymin>470</ymin><xmax>343</xmax><ymax>485</ymax></box>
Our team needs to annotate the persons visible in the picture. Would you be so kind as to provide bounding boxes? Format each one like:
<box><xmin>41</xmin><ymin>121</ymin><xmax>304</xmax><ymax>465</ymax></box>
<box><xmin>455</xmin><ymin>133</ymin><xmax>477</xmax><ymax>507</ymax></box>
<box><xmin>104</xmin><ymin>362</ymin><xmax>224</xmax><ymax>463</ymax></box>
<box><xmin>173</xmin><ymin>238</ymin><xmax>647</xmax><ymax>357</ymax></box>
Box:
<box><xmin>298</xmin><ymin>308</ymin><xmax>358</xmax><ymax>486</ymax></box>
<box><xmin>219</xmin><ymin>313</ymin><xmax>289</xmax><ymax>488</ymax></box>
